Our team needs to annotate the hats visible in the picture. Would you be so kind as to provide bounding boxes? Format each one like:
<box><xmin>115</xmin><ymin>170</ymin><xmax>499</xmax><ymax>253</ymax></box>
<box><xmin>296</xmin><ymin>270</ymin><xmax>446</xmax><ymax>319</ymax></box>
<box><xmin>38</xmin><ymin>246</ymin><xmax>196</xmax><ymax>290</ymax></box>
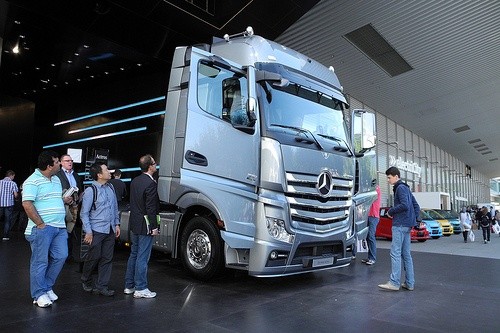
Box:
<box><xmin>461</xmin><ymin>206</ymin><xmax>467</xmax><ymax>214</ymax></box>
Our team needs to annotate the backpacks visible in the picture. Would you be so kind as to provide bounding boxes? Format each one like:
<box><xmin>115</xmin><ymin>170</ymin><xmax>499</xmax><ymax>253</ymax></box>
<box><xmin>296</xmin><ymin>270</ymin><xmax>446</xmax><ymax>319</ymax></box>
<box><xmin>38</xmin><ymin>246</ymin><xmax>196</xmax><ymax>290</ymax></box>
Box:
<box><xmin>75</xmin><ymin>180</ymin><xmax>116</xmax><ymax>229</ymax></box>
<box><xmin>479</xmin><ymin>212</ymin><xmax>491</xmax><ymax>229</ymax></box>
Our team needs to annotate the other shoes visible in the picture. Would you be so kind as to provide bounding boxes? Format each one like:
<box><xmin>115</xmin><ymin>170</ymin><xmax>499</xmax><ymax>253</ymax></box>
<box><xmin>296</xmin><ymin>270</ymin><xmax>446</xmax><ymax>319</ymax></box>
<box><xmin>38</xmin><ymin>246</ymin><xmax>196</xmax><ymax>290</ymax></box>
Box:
<box><xmin>2</xmin><ymin>234</ymin><xmax>13</xmax><ymax>241</ymax></box>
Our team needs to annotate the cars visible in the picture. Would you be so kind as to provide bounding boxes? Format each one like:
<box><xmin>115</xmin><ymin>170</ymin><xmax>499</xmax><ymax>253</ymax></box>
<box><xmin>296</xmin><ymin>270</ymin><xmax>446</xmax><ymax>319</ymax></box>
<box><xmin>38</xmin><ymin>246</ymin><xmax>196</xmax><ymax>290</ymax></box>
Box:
<box><xmin>422</xmin><ymin>208</ymin><xmax>454</xmax><ymax>236</ymax></box>
<box><xmin>445</xmin><ymin>210</ymin><xmax>462</xmax><ymax>222</ymax></box>
<box><xmin>433</xmin><ymin>208</ymin><xmax>462</xmax><ymax>235</ymax></box>
<box><xmin>375</xmin><ymin>207</ymin><xmax>430</xmax><ymax>243</ymax></box>
<box><xmin>418</xmin><ymin>208</ymin><xmax>444</xmax><ymax>239</ymax></box>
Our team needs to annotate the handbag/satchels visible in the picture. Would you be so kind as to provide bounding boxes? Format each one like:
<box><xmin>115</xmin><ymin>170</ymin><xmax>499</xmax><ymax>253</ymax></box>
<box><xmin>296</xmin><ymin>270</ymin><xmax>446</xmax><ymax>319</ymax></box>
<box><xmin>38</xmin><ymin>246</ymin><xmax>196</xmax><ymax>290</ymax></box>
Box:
<box><xmin>467</xmin><ymin>231</ymin><xmax>476</xmax><ymax>242</ymax></box>
<box><xmin>463</xmin><ymin>213</ymin><xmax>473</xmax><ymax>230</ymax></box>
<box><xmin>491</xmin><ymin>221</ymin><xmax>500</xmax><ymax>234</ymax></box>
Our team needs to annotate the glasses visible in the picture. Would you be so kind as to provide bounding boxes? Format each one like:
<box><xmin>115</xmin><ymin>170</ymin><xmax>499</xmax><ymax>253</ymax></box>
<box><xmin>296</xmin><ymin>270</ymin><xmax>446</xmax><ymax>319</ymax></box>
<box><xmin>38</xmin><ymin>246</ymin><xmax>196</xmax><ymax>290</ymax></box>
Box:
<box><xmin>62</xmin><ymin>159</ymin><xmax>74</xmax><ymax>163</ymax></box>
<box><xmin>151</xmin><ymin>161</ymin><xmax>158</xmax><ymax>167</ymax></box>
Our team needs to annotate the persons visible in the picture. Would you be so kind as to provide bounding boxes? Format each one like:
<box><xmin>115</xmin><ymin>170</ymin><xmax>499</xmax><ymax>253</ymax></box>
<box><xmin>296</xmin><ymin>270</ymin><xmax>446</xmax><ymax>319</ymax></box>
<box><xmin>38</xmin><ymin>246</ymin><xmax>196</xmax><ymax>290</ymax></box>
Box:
<box><xmin>361</xmin><ymin>184</ymin><xmax>380</xmax><ymax>263</ymax></box>
<box><xmin>108</xmin><ymin>168</ymin><xmax>125</xmax><ymax>227</ymax></box>
<box><xmin>22</xmin><ymin>150</ymin><xmax>70</xmax><ymax>307</ymax></box>
<box><xmin>54</xmin><ymin>154</ymin><xmax>83</xmax><ymax>263</ymax></box>
<box><xmin>80</xmin><ymin>161</ymin><xmax>122</xmax><ymax>295</ymax></box>
<box><xmin>0</xmin><ymin>171</ymin><xmax>20</xmax><ymax>241</ymax></box>
<box><xmin>378</xmin><ymin>167</ymin><xmax>421</xmax><ymax>291</ymax></box>
<box><xmin>459</xmin><ymin>204</ymin><xmax>500</xmax><ymax>243</ymax></box>
<box><xmin>121</xmin><ymin>153</ymin><xmax>161</xmax><ymax>298</ymax></box>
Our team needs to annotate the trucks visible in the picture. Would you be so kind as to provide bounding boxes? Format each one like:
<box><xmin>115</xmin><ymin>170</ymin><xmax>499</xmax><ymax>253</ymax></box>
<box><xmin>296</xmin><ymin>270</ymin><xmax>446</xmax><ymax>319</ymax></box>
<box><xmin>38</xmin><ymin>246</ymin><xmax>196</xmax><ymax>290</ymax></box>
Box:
<box><xmin>66</xmin><ymin>26</ymin><xmax>378</xmax><ymax>287</ymax></box>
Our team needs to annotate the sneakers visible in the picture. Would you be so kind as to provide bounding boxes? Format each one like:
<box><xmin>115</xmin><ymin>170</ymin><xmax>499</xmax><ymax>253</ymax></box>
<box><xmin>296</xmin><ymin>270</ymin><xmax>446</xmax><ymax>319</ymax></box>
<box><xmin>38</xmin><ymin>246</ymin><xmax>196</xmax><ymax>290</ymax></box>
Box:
<box><xmin>124</xmin><ymin>286</ymin><xmax>136</xmax><ymax>295</ymax></box>
<box><xmin>47</xmin><ymin>289</ymin><xmax>59</xmax><ymax>302</ymax></box>
<box><xmin>82</xmin><ymin>283</ymin><xmax>93</xmax><ymax>292</ymax></box>
<box><xmin>362</xmin><ymin>256</ymin><xmax>370</xmax><ymax>263</ymax></box>
<box><xmin>402</xmin><ymin>281</ymin><xmax>414</xmax><ymax>290</ymax></box>
<box><xmin>366</xmin><ymin>260</ymin><xmax>374</xmax><ymax>265</ymax></box>
<box><xmin>31</xmin><ymin>293</ymin><xmax>53</xmax><ymax>308</ymax></box>
<box><xmin>90</xmin><ymin>286</ymin><xmax>117</xmax><ymax>297</ymax></box>
<box><xmin>131</xmin><ymin>288</ymin><xmax>158</xmax><ymax>299</ymax></box>
<box><xmin>377</xmin><ymin>281</ymin><xmax>400</xmax><ymax>291</ymax></box>
